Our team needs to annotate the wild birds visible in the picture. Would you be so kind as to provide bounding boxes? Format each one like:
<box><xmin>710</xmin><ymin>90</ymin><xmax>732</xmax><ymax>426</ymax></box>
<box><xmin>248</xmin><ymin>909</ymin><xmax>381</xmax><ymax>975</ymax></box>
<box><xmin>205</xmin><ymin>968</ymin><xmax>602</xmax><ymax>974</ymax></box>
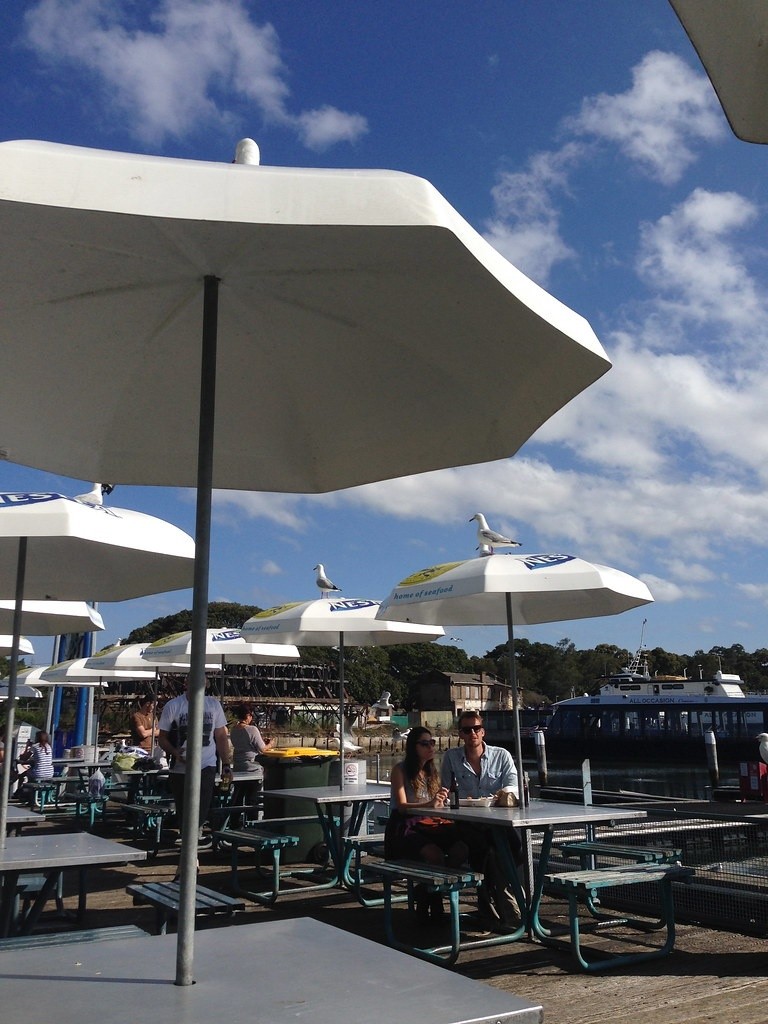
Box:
<box><xmin>371</xmin><ymin>692</ymin><xmax>395</xmax><ymax>710</ymax></box>
<box><xmin>114</xmin><ymin>638</ymin><xmax>122</xmax><ymax>648</ymax></box>
<box><xmin>329</xmin><ymin>738</ymin><xmax>365</xmax><ymax>759</ymax></box>
<box><xmin>756</xmin><ymin>733</ymin><xmax>768</xmax><ymax>765</ymax></box>
<box><xmin>450</xmin><ymin>637</ymin><xmax>463</xmax><ymax>642</ymax></box>
<box><xmin>313</xmin><ymin>563</ymin><xmax>342</xmax><ymax>599</ymax></box>
<box><xmin>73</xmin><ymin>481</ymin><xmax>114</xmax><ymax>505</ymax></box>
<box><xmin>391</xmin><ymin>727</ymin><xmax>411</xmax><ymax>741</ymax></box>
<box><xmin>468</xmin><ymin>512</ymin><xmax>522</xmax><ymax>558</ymax></box>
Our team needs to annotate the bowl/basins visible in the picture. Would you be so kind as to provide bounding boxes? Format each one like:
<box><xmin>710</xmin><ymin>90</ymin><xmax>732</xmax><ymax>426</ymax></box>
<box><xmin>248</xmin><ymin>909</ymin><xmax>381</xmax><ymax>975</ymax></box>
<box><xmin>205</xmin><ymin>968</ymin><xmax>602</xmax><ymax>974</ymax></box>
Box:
<box><xmin>459</xmin><ymin>796</ymin><xmax>498</xmax><ymax>807</ymax></box>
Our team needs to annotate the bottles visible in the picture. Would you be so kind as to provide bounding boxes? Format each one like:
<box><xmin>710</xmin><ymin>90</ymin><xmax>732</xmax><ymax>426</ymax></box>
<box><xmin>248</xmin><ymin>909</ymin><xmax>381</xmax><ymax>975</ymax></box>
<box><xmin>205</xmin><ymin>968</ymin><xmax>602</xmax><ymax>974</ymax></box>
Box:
<box><xmin>449</xmin><ymin>772</ymin><xmax>460</xmax><ymax>809</ymax></box>
<box><xmin>221</xmin><ymin>769</ymin><xmax>230</xmax><ymax>791</ymax></box>
<box><xmin>518</xmin><ymin>770</ymin><xmax>529</xmax><ymax>807</ymax></box>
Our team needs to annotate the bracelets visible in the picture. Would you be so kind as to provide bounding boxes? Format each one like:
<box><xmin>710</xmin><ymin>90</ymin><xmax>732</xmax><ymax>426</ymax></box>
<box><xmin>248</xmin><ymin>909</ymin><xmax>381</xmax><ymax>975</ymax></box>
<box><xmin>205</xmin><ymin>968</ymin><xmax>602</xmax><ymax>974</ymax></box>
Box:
<box><xmin>222</xmin><ymin>764</ymin><xmax>230</xmax><ymax>769</ymax></box>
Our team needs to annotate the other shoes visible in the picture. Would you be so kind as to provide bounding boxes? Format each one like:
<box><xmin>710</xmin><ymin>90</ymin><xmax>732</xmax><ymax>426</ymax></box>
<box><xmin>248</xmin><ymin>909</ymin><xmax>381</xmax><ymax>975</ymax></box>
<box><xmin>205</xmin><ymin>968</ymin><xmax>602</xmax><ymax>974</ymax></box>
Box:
<box><xmin>476</xmin><ymin>902</ymin><xmax>500</xmax><ymax>920</ymax></box>
<box><xmin>496</xmin><ymin>898</ymin><xmax>518</xmax><ymax>921</ymax></box>
<box><xmin>415</xmin><ymin>896</ymin><xmax>429</xmax><ymax>920</ymax></box>
<box><xmin>198</xmin><ymin>835</ymin><xmax>213</xmax><ymax>849</ymax></box>
<box><xmin>430</xmin><ymin>893</ymin><xmax>443</xmax><ymax>916</ymax></box>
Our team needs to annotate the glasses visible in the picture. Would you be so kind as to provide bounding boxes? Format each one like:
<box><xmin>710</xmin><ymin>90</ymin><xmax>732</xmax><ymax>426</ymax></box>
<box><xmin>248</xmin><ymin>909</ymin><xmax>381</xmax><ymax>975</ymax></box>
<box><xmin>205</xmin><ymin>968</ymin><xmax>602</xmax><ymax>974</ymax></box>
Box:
<box><xmin>415</xmin><ymin>739</ymin><xmax>435</xmax><ymax>748</ymax></box>
<box><xmin>250</xmin><ymin>713</ymin><xmax>255</xmax><ymax>719</ymax></box>
<box><xmin>460</xmin><ymin>725</ymin><xmax>483</xmax><ymax>735</ymax></box>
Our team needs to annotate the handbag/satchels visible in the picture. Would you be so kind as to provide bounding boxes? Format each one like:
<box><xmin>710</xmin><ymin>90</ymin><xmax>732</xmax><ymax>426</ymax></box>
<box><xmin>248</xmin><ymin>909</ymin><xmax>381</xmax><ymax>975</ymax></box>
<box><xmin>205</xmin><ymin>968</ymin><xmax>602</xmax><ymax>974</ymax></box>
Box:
<box><xmin>87</xmin><ymin>767</ymin><xmax>106</xmax><ymax>800</ymax></box>
<box><xmin>165</xmin><ymin>721</ymin><xmax>181</xmax><ymax>769</ymax></box>
<box><xmin>112</xmin><ymin>745</ymin><xmax>172</xmax><ymax>771</ymax></box>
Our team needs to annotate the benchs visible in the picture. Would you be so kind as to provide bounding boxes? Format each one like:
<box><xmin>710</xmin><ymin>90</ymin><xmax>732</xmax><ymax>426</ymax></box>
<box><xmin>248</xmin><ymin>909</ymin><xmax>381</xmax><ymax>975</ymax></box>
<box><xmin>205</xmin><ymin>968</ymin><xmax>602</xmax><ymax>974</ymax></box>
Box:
<box><xmin>0</xmin><ymin>772</ymin><xmax>693</xmax><ymax>951</ymax></box>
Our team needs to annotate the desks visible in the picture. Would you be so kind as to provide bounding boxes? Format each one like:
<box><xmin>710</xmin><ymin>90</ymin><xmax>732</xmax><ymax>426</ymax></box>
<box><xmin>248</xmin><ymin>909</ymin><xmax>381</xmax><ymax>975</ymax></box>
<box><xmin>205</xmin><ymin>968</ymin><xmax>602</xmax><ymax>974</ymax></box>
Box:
<box><xmin>0</xmin><ymin>833</ymin><xmax>147</xmax><ymax>937</ymax></box>
<box><xmin>0</xmin><ymin>918</ymin><xmax>546</xmax><ymax>1023</ymax></box>
<box><xmin>213</xmin><ymin>771</ymin><xmax>264</xmax><ymax>804</ymax></box>
<box><xmin>263</xmin><ymin>785</ymin><xmax>393</xmax><ymax>897</ymax></box>
<box><xmin>18</xmin><ymin>758</ymin><xmax>84</xmax><ymax>777</ymax></box>
<box><xmin>411</xmin><ymin>802</ymin><xmax>647</xmax><ymax>951</ymax></box>
<box><xmin>6</xmin><ymin>806</ymin><xmax>44</xmax><ymax>838</ymax></box>
<box><xmin>124</xmin><ymin>768</ymin><xmax>169</xmax><ymax>805</ymax></box>
<box><xmin>54</xmin><ymin>763</ymin><xmax>113</xmax><ymax>793</ymax></box>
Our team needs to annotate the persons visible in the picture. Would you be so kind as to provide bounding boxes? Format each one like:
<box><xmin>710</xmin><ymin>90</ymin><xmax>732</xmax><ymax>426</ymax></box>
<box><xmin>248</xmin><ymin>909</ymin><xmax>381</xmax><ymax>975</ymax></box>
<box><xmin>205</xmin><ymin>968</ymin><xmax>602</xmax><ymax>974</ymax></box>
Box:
<box><xmin>0</xmin><ymin>729</ymin><xmax>53</xmax><ymax>805</ymax></box>
<box><xmin>230</xmin><ymin>704</ymin><xmax>274</xmax><ymax>827</ymax></box>
<box><xmin>157</xmin><ymin>671</ymin><xmax>233</xmax><ymax>883</ymax></box>
<box><xmin>389</xmin><ymin>712</ymin><xmax>522</xmax><ymax>930</ymax></box>
<box><xmin>126</xmin><ymin>695</ymin><xmax>160</xmax><ymax>804</ymax></box>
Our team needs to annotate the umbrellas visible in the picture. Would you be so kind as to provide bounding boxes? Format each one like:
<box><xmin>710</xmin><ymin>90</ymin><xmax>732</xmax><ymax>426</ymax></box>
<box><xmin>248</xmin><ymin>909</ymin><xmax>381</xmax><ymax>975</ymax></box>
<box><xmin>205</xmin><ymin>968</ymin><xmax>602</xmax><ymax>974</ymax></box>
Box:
<box><xmin>0</xmin><ymin>666</ymin><xmax>108</xmax><ymax>734</ymax></box>
<box><xmin>142</xmin><ymin>628</ymin><xmax>301</xmax><ymax>705</ymax></box>
<box><xmin>84</xmin><ymin>643</ymin><xmax>222</xmax><ymax>756</ymax></box>
<box><xmin>375</xmin><ymin>554</ymin><xmax>655</xmax><ymax>810</ymax></box>
<box><xmin>40</xmin><ymin>657</ymin><xmax>160</xmax><ymax>763</ymax></box>
<box><xmin>239</xmin><ymin>597</ymin><xmax>446</xmax><ymax>791</ymax></box>
<box><xmin>0</xmin><ymin>491</ymin><xmax>195</xmax><ymax>848</ymax></box>
<box><xmin>0</xmin><ymin>600</ymin><xmax>107</xmax><ymax>697</ymax></box>
<box><xmin>0</xmin><ymin>136</ymin><xmax>614</xmax><ymax>1001</ymax></box>
